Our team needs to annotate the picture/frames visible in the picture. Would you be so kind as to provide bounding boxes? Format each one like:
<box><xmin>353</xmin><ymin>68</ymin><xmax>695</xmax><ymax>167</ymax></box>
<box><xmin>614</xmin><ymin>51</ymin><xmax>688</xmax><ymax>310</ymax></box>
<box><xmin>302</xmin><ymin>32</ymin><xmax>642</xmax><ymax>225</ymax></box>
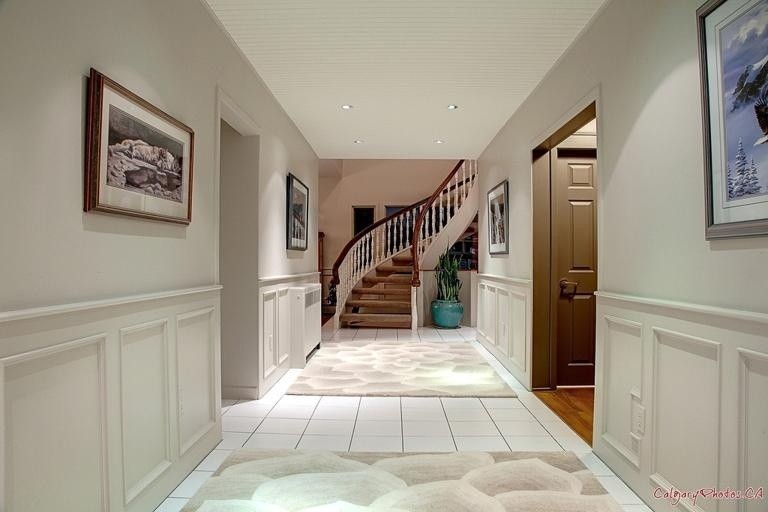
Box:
<box><xmin>696</xmin><ymin>0</ymin><xmax>768</xmax><ymax>240</ymax></box>
<box><xmin>286</xmin><ymin>172</ymin><xmax>310</xmax><ymax>251</ymax></box>
<box><xmin>83</xmin><ymin>67</ymin><xmax>195</xmax><ymax>226</ymax></box>
<box><xmin>486</xmin><ymin>179</ymin><xmax>509</xmax><ymax>255</ymax></box>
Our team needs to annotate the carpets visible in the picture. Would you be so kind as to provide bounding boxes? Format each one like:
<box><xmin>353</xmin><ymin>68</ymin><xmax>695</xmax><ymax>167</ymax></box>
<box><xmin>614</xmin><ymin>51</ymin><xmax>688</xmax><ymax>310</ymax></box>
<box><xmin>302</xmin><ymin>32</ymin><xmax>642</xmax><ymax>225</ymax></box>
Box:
<box><xmin>284</xmin><ymin>340</ymin><xmax>519</xmax><ymax>399</ymax></box>
<box><xmin>180</xmin><ymin>450</ymin><xmax>623</xmax><ymax>512</ymax></box>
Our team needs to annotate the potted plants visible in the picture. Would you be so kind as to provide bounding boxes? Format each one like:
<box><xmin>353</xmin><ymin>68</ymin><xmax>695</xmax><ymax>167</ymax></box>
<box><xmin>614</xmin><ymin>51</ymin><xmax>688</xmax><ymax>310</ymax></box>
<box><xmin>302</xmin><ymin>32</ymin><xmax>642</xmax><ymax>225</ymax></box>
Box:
<box><xmin>430</xmin><ymin>240</ymin><xmax>464</xmax><ymax>329</ymax></box>
<box><xmin>326</xmin><ymin>278</ymin><xmax>340</xmax><ymax>313</ymax></box>
